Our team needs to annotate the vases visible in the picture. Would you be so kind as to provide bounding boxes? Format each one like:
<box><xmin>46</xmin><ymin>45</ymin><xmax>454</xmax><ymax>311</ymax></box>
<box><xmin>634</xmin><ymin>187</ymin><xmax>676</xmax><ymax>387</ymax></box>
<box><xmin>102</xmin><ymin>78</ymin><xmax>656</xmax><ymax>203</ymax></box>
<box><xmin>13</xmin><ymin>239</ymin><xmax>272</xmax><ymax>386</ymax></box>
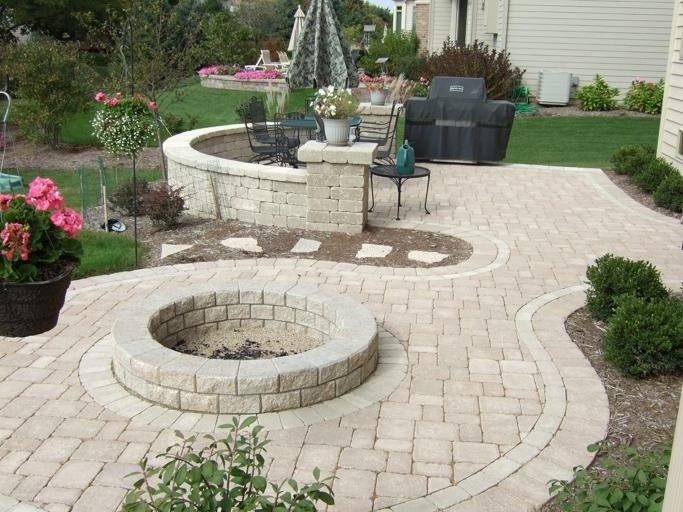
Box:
<box><xmin>0</xmin><ymin>262</ymin><xmax>74</xmax><ymax>337</ymax></box>
<box><xmin>369</xmin><ymin>88</ymin><xmax>387</xmax><ymax>106</ymax></box>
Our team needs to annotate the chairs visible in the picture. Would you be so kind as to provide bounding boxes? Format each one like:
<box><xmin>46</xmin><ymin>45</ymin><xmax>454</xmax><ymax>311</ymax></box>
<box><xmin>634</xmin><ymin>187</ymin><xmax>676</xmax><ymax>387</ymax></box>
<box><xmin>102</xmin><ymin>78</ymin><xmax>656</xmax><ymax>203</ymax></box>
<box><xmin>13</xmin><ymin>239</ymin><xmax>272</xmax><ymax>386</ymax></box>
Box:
<box><xmin>233</xmin><ymin>96</ymin><xmax>409</xmax><ymax>193</ymax></box>
<box><xmin>243</xmin><ymin>49</ymin><xmax>290</xmax><ymax>76</ymax></box>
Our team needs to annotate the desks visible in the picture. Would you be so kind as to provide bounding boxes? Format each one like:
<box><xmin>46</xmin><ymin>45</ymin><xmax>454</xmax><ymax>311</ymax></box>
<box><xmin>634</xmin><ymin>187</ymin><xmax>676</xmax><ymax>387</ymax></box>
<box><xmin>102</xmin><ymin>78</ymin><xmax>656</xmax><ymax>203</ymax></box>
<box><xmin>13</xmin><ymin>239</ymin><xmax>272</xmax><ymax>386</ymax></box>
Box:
<box><xmin>366</xmin><ymin>163</ymin><xmax>431</xmax><ymax>222</ymax></box>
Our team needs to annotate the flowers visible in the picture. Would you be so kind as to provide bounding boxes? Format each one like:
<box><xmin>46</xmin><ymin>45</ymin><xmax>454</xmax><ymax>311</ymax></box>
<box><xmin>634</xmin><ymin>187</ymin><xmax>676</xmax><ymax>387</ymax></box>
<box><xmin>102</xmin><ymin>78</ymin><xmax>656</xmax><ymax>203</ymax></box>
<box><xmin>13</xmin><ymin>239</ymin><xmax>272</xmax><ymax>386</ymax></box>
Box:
<box><xmin>358</xmin><ymin>71</ymin><xmax>390</xmax><ymax>92</ymax></box>
<box><xmin>87</xmin><ymin>88</ymin><xmax>159</xmax><ymax>164</ymax></box>
<box><xmin>0</xmin><ymin>172</ymin><xmax>85</xmax><ymax>285</ymax></box>
<box><xmin>308</xmin><ymin>82</ymin><xmax>362</xmax><ymax>120</ymax></box>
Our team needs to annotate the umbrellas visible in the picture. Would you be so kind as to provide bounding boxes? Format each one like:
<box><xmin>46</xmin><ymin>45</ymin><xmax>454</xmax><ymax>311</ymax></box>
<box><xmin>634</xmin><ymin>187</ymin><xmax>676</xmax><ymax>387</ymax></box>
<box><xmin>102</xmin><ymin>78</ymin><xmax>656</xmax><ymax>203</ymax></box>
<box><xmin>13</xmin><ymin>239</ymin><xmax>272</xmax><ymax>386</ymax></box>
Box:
<box><xmin>280</xmin><ymin>0</ymin><xmax>362</xmax><ymax>94</ymax></box>
<box><xmin>285</xmin><ymin>3</ymin><xmax>305</xmax><ymax>64</ymax></box>
